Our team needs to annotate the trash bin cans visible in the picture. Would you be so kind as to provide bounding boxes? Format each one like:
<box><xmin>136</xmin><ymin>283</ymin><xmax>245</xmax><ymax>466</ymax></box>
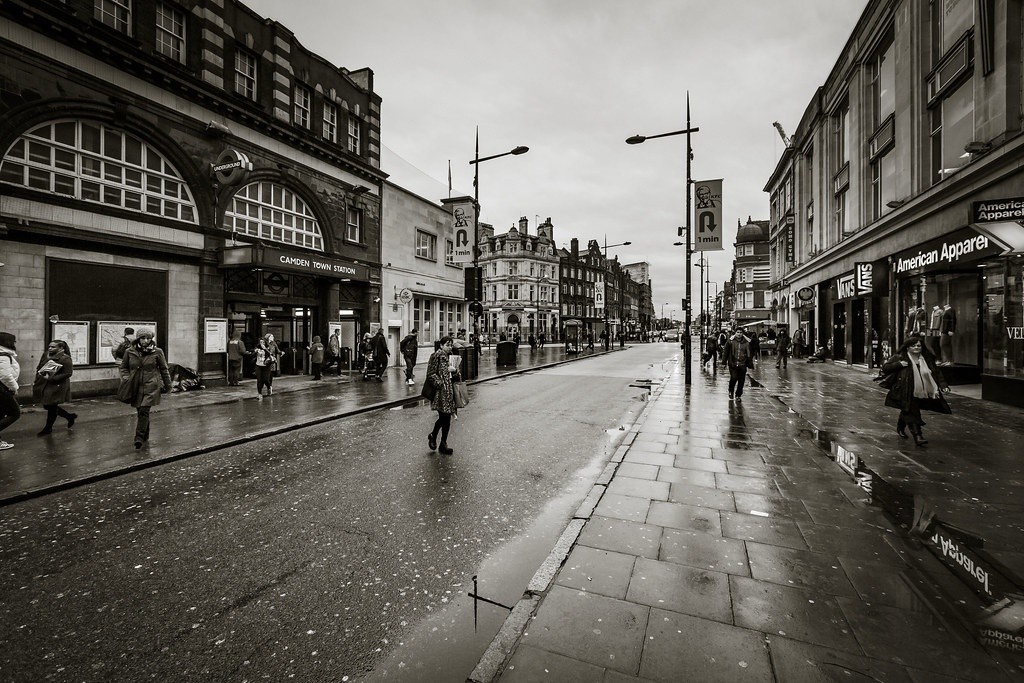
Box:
<box><xmin>434</xmin><ymin>340</ymin><xmax>465</xmax><ymax>381</ymax></box>
<box><xmin>495</xmin><ymin>341</ymin><xmax>517</xmax><ymax>368</ymax></box>
<box><xmin>453</xmin><ymin>338</ymin><xmax>476</xmax><ymax>380</ymax></box>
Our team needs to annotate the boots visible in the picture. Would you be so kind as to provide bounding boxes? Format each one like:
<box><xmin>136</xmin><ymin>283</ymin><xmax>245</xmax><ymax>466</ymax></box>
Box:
<box><xmin>896</xmin><ymin>418</ymin><xmax>908</xmax><ymax>438</ymax></box>
<box><xmin>909</xmin><ymin>422</ymin><xmax>928</xmax><ymax>445</ymax></box>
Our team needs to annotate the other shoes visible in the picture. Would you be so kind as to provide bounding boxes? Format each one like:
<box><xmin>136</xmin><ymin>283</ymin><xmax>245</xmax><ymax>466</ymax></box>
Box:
<box><xmin>375</xmin><ymin>375</ymin><xmax>384</xmax><ymax>382</ymax></box>
<box><xmin>257</xmin><ymin>394</ymin><xmax>263</xmax><ymax>399</ymax></box>
<box><xmin>728</xmin><ymin>389</ymin><xmax>733</xmax><ymax>399</ymax></box>
<box><xmin>713</xmin><ymin>363</ymin><xmax>717</xmax><ymax>366</ymax></box>
<box><xmin>134</xmin><ymin>438</ymin><xmax>143</xmax><ymax>449</ymax></box>
<box><xmin>229</xmin><ymin>380</ymin><xmax>243</xmax><ymax>385</ymax></box>
<box><xmin>268</xmin><ymin>386</ymin><xmax>272</xmax><ymax>396</ymax></box>
<box><xmin>408</xmin><ymin>378</ymin><xmax>415</xmax><ymax>385</ymax></box>
<box><xmin>428</xmin><ymin>433</ymin><xmax>436</xmax><ymax>450</ymax></box>
<box><xmin>38</xmin><ymin>424</ymin><xmax>52</xmax><ymax>436</ymax></box>
<box><xmin>439</xmin><ymin>441</ymin><xmax>453</xmax><ymax>454</ymax></box>
<box><xmin>703</xmin><ymin>361</ymin><xmax>706</xmax><ymax>366</ymax></box>
<box><xmin>312</xmin><ymin>375</ymin><xmax>321</xmax><ymax>380</ymax></box>
<box><xmin>0</xmin><ymin>440</ymin><xmax>15</xmax><ymax>451</ymax></box>
<box><xmin>405</xmin><ymin>381</ymin><xmax>409</xmax><ymax>386</ymax></box>
<box><xmin>67</xmin><ymin>413</ymin><xmax>77</xmax><ymax>428</ymax></box>
<box><xmin>735</xmin><ymin>394</ymin><xmax>741</xmax><ymax>402</ymax></box>
<box><xmin>776</xmin><ymin>364</ymin><xmax>787</xmax><ymax>369</ymax></box>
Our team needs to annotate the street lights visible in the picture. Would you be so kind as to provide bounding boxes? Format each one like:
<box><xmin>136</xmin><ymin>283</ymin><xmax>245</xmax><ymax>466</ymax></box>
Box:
<box><xmin>662</xmin><ymin>302</ymin><xmax>668</xmax><ymax>329</ymax></box>
<box><xmin>626</xmin><ymin>128</ymin><xmax>690</xmax><ymax>385</ymax></box>
<box><xmin>694</xmin><ymin>258</ymin><xmax>708</xmax><ymax>338</ymax></box>
<box><xmin>673</xmin><ymin>243</ymin><xmax>705</xmax><ymax>360</ymax></box>
<box><xmin>604</xmin><ymin>242</ymin><xmax>632</xmax><ymax>350</ymax></box>
<box><xmin>705</xmin><ymin>280</ymin><xmax>717</xmax><ymax>329</ymax></box>
<box><xmin>474</xmin><ymin>146</ymin><xmax>528</xmax><ymax>347</ymax></box>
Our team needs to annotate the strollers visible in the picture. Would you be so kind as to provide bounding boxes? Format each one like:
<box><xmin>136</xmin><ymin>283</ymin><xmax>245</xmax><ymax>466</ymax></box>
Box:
<box><xmin>363</xmin><ymin>349</ymin><xmax>379</xmax><ymax>381</ymax></box>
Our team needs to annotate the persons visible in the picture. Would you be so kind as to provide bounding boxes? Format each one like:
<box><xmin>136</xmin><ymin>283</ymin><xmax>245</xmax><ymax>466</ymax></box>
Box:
<box><xmin>400</xmin><ymin>329</ymin><xmax>418</xmax><ymax>385</ymax></box>
<box><xmin>775</xmin><ymin>329</ymin><xmax>790</xmax><ymax>369</ymax></box>
<box><xmin>119</xmin><ymin>327</ymin><xmax>172</xmax><ymax>449</ymax></box>
<box><xmin>651</xmin><ymin>332</ymin><xmax>654</xmax><ymax>342</ymax></box>
<box><xmin>33</xmin><ymin>340</ymin><xmax>77</xmax><ymax>437</ymax></box>
<box><xmin>882</xmin><ymin>337</ymin><xmax>951</xmax><ymax>445</ymax></box>
<box><xmin>500</xmin><ymin>332</ymin><xmax>545</xmax><ymax>354</ymax></box>
<box><xmin>111</xmin><ymin>327</ymin><xmax>136</xmax><ymax>386</ymax></box>
<box><xmin>704</xmin><ymin>330</ymin><xmax>735</xmax><ymax>367</ymax></box>
<box><xmin>457</xmin><ymin>328</ymin><xmax>482</xmax><ymax>355</ymax></box>
<box><xmin>0</xmin><ymin>332</ymin><xmax>20</xmax><ymax>449</ymax></box>
<box><xmin>324</xmin><ymin>329</ymin><xmax>343</xmax><ymax>376</ymax></box>
<box><xmin>807</xmin><ymin>346</ymin><xmax>825</xmax><ymax>362</ymax></box>
<box><xmin>722</xmin><ymin>327</ymin><xmax>752</xmax><ymax>402</ymax></box>
<box><xmin>793</xmin><ymin>328</ymin><xmax>804</xmax><ymax>359</ymax></box>
<box><xmin>658</xmin><ymin>331</ymin><xmax>664</xmax><ymax>342</ymax></box>
<box><xmin>367</xmin><ymin>328</ymin><xmax>390</xmax><ymax>382</ymax></box>
<box><xmin>227</xmin><ymin>331</ymin><xmax>251</xmax><ymax>386</ymax></box>
<box><xmin>421</xmin><ymin>336</ymin><xmax>461</xmax><ymax>454</ymax></box>
<box><xmin>264</xmin><ymin>333</ymin><xmax>285</xmax><ymax>364</ymax></box>
<box><xmin>908</xmin><ymin>305</ymin><xmax>956</xmax><ymax>366</ymax></box>
<box><xmin>584</xmin><ymin>329</ymin><xmax>624</xmax><ymax>351</ymax></box>
<box><xmin>308</xmin><ymin>336</ymin><xmax>324</xmax><ymax>380</ymax></box>
<box><xmin>744</xmin><ymin>329</ymin><xmax>777</xmax><ymax>368</ymax></box>
<box><xmin>250</xmin><ymin>338</ymin><xmax>272</xmax><ymax>400</ymax></box>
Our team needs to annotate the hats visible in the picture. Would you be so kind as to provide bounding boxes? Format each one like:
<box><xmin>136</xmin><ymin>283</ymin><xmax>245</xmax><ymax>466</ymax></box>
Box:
<box><xmin>136</xmin><ymin>327</ymin><xmax>155</xmax><ymax>339</ymax></box>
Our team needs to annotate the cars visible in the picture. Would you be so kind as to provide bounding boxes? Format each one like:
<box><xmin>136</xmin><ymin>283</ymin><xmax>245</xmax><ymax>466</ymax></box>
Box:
<box><xmin>646</xmin><ymin>329</ymin><xmax>686</xmax><ymax>342</ymax></box>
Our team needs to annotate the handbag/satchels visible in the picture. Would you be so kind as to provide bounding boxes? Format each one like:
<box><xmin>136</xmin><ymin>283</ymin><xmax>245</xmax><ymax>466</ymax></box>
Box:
<box><xmin>453</xmin><ymin>381</ymin><xmax>469</xmax><ymax>408</ymax></box>
<box><xmin>746</xmin><ymin>357</ymin><xmax>754</xmax><ymax>370</ymax></box>
<box><xmin>37</xmin><ymin>359</ymin><xmax>63</xmax><ymax>378</ymax></box>
<box><xmin>116</xmin><ymin>369</ymin><xmax>140</xmax><ymax>403</ymax></box>
<box><xmin>325</xmin><ymin>343</ymin><xmax>334</xmax><ymax>357</ymax></box>
<box><xmin>873</xmin><ymin>363</ymin><xmax>896</xmax><ymax>389</ymax></box>
<box><xmin>421</xmin><ymin>378</ymin><xmax>436</xmax><ymax>402</ymax></box>
<box><xmin>372</xmin><ymin>347</ymin><xmax>378</xmax><ymax>362</ymax></box>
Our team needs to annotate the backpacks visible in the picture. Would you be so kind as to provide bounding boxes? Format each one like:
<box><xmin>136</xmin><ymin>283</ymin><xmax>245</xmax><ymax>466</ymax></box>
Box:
<box><xmin>116</xmin><ymin>335</ymin><xmax>130</xmax><ymax>359</ymax></box>
